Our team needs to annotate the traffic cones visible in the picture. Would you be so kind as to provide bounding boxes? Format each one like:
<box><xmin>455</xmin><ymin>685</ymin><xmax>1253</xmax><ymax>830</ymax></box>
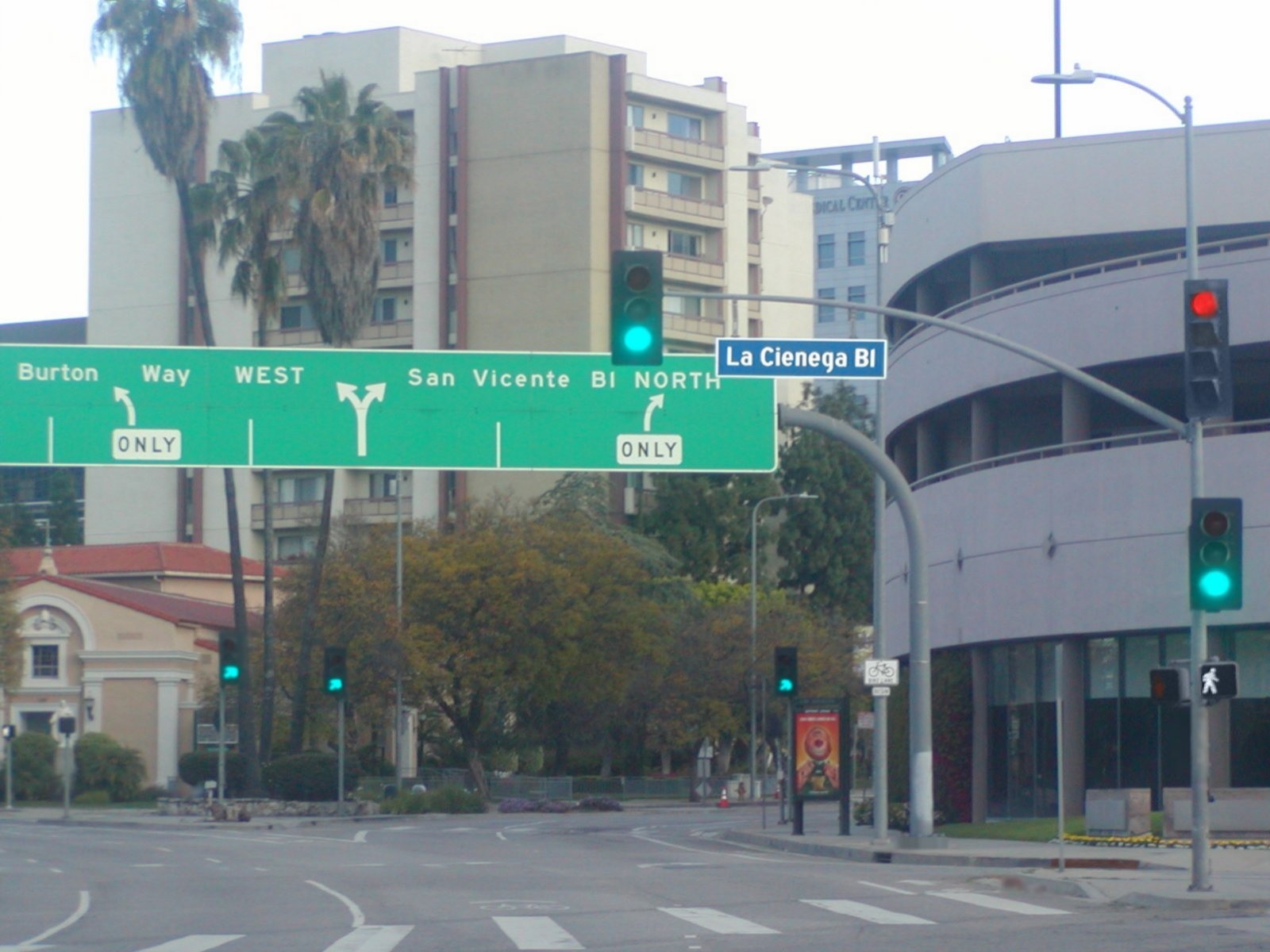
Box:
<box><xmin>775</xmin><ymin>782</ymin><xmax>781</xmax><ymax>800</ymax></box>
<box><xmin>717</xmin><ymin>788</ymin><xmax>730</xmax><ymax>807</ymax></box>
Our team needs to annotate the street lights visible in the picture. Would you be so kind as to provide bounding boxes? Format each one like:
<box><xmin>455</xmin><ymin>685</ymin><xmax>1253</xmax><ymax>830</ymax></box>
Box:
<box><xmin>1030</xmin><ymin>62</ymin><xmax>1214</xmax><ymax>893</ymax></box>
<box><xmin>749</xmin><ymin>491</ymin><xmax>819</xmax><ymax>799</ymax></box>
<box><xmin>727</xmin><ymin>159</ymin><xmax>891</xmax><ymax>846</ymax></box>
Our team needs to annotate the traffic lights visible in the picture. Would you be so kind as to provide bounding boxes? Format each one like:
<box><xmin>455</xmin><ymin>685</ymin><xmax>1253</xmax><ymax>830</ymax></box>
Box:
<box><xmin>1187</xmin><ymin>495</ymin><xmax>1243</xmax><ymax>613</ymax></box>
<box><xmin>218</xmin><ymin>629</ymin><xmax>241</xmax><ymax>685</ymax></box>
<box><xmin>1198</xmin><ymin>661</ymin><xmax>1238</xmax><ymax>699</ymax></box>
<box><xmin>1149</xmin><ymin>666</ymin><xmax>1189</xmax><ymax>702</ymax></box>
<box><xmin>610</xmin><ymin>249</ymin><xmax>664</xmax><ymax>365</ymax></box>
<box><xmin>1183</xmin><ymin>279</ymin><xmax>1233</xmax><ymax>420</ymax></box>
<box><xmin>324</xmin><ymin>646</ymin><xmax>347</xmax><ymax>694</ymax></box>
<box><xmin>774</xmin><ymin>646</ymin><xmax>798</xmax><ymax>694</ymax></box>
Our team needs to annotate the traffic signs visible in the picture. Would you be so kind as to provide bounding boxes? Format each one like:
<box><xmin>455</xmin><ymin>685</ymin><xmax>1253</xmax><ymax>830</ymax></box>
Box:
<box><xmin>0</xmin><ymin>343</ymin><xmax>779</xmax><ymax>474</ymax></box>
